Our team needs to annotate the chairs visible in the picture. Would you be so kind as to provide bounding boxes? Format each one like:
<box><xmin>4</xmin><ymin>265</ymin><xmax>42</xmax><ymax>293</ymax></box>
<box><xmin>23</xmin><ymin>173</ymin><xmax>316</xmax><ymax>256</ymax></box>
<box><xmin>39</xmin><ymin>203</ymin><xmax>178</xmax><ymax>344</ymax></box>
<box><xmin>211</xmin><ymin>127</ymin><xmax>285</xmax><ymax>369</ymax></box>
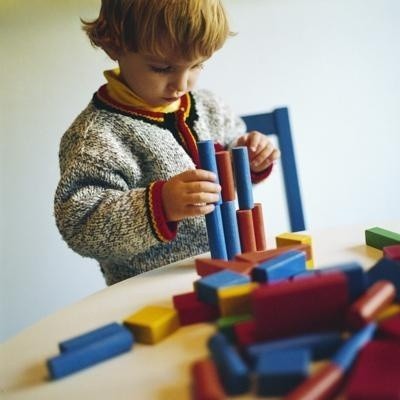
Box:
<box><xmin>185</xmin><ymin>108</ymin><xmax>329</xmax><ymax>233</ymax></box>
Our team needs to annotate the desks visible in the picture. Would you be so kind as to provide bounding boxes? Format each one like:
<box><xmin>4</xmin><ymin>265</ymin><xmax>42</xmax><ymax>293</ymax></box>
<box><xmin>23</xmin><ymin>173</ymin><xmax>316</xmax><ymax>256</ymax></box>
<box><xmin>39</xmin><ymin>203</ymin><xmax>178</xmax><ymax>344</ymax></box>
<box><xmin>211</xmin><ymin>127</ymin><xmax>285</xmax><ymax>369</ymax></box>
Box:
<box><xmin>1</xmin><ymin>226</ymin><xmax>399</xmax><ymax>399</ymax></box>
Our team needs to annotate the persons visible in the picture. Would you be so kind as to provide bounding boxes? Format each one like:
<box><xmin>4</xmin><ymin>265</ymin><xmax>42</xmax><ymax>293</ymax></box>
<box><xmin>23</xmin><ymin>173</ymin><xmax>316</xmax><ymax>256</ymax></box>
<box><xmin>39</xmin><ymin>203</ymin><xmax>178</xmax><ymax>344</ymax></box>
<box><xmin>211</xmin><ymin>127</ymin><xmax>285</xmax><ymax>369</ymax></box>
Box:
<box><xmin>52</xmin><ymin>0</ymin><xmax>283</xmax><ymax>287</ymax></box>
